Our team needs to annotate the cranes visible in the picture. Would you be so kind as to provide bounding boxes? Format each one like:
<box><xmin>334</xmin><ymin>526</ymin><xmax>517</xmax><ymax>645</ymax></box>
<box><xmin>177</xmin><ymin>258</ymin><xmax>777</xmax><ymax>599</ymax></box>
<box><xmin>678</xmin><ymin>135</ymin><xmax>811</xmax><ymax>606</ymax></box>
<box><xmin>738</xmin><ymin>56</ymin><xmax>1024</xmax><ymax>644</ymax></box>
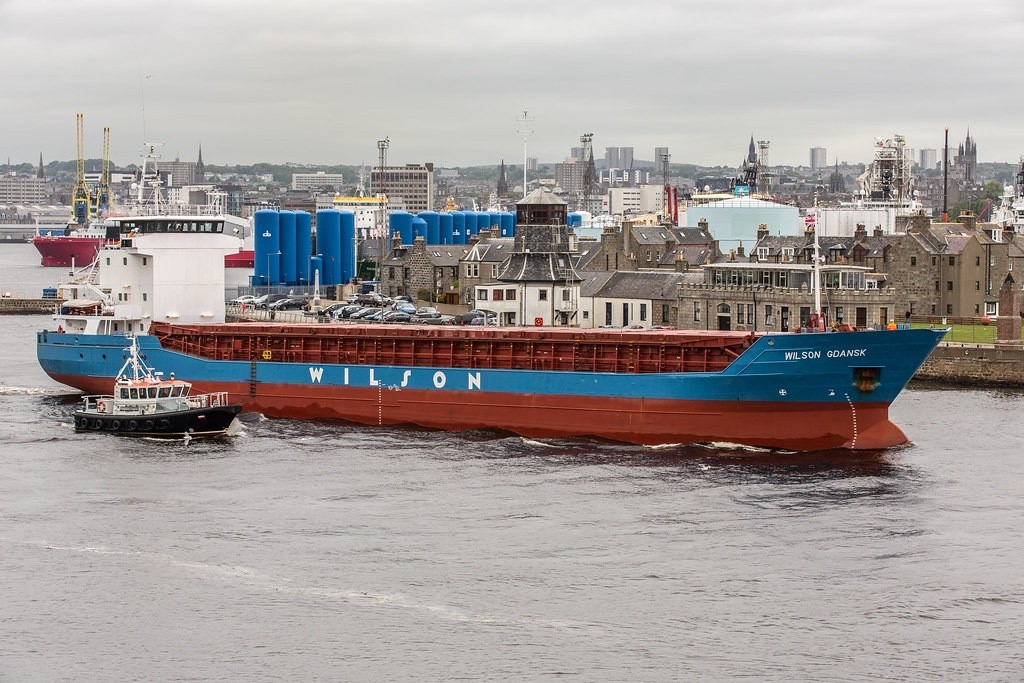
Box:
<box><xmin>90</xmin><ymin>127</ymin><xmax>115</xmax><ymax>221</ymax></box>
<box><xmin>64</xmin><ymin>114</ymin><xmax>91</xmax><ymax>236</ymax></box>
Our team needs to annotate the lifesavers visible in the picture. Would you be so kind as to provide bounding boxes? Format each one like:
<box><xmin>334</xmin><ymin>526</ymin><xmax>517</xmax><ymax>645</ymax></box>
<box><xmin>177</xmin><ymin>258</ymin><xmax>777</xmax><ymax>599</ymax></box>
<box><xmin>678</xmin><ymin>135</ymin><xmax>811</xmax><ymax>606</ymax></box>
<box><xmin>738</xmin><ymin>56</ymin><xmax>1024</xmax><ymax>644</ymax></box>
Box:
<box><xmin>130</xmin><ymin>421</ymin><xmax>137</xmax><ymax>429</ymax></box>
<box><xmin>161</xmin><ymin>420</ymin><xmax>170</xmax><ymax>429</ymax></box>
<box><xmin>96</xmin><ymin>420</ymin><xmax>102</xmax><ymax>429</ymax></box>
<box><xmin>113</xmin><ymin>421</ymin><xmax>121</xmax><ymax>429</ymax></box>
<box><xmin>98</xmin><ymin>402</ymin><xmax>106</xmax><ymax>411</ymax></box>
<box><xmin>81</xmin><ymin>419</ymin><xmax>88</xmax><ymax>428</ymax></box>
<box><xmin>146</xmin><ymin>421</ymin><xmax>154</xmax><ymax>429</ymax></box>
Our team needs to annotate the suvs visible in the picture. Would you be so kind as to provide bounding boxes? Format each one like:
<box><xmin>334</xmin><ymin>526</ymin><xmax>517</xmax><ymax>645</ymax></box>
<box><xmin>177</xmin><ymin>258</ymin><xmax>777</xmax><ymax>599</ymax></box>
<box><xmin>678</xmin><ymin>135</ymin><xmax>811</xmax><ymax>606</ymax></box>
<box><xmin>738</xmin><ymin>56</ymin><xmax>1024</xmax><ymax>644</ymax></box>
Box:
<box><xmin>358</xmin><ymin>295</ymin><xmax>387</xmax><ymax>307</ymax></box>
<box><xmin>246</xmin><ymin>293</ymin><xmax>285</xmax><ymax>309</ymax></box>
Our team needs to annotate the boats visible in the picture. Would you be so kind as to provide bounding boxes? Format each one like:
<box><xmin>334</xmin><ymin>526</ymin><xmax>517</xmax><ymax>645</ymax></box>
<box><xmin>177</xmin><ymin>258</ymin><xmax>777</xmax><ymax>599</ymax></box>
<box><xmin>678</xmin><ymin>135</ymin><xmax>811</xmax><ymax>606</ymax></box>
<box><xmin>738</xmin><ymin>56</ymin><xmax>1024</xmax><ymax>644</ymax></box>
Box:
<box><xmin>33</xmin><ymin>228</ymin><xmax>107</xmax><ymax>267</ymax></box>
<box><xmin>73</xmin><ymin>333</ymin><xmax>242</xmax><ymax>439</ymax></box>
<box><xmin>37</xmin><ymin>254</ymin><xmax>951</xmax><ymax>452</ymax></box>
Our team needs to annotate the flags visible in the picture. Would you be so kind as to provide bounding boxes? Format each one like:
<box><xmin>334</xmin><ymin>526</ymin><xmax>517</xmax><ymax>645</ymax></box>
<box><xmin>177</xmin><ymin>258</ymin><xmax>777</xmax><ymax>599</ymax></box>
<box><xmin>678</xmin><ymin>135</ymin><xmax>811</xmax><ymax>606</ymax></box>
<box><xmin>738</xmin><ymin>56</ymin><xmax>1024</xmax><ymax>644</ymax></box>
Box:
<box><xmin>806</xmin><ymin>217</ymin><xmax>816</xmax><ymax>226</ymax></box>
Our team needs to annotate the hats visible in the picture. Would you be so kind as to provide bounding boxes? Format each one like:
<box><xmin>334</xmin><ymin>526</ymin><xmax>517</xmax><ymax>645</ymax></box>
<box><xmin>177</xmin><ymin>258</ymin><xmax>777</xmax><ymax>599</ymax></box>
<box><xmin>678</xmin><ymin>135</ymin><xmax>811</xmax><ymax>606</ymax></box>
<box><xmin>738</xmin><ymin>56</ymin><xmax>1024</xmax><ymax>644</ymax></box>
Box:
<box><xmin>889</xmin><ymin>318</ymin><xmax>894</xmax><ymax>323</ymax></box>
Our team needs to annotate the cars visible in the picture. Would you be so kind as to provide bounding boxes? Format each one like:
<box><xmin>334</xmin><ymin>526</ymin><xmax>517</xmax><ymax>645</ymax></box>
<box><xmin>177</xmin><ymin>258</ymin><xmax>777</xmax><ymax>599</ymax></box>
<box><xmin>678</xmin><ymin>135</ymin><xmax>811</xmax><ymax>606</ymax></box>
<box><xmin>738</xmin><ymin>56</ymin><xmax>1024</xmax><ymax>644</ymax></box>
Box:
<box><xmin>392</xmin><ymin>301</ymin><xmax>416</xmax><ymax>314</ymax></box>
<box><xmin>390</xmin><ymin>296</ymin><xmax>412</xmax><ymax>303</ymax></box>
<box><xmin>455</xmin><ymin>308</ymin><xmax>498</xmax><ymax>325</ymax></box>
<box><xmin>271</xmin><ymin>298</ymin><xmax>310</xmax><ymax>311</ymax></box>
<box><xmin>230</xmin><ymin>295</ymin><xmax>259</xmax><ymax>306</ymax></box>
<box><xmin>410</xmin><ymin>314</ymin><xmax>445</xmax><ymax>325</ymax></box>
<box><xmin>415</xmin><ymin>307</ymin><xmax>441</xmax><ymax>318</ymax></box>
<box><xmin>322</xmin><ymin>303</ymin><xmax>412</xmax><ymax>322</ymax></box>
<box><xmin>346</xmin><ymin>294</ymin><xmax>361</xmax><ymax>303</ymax></box>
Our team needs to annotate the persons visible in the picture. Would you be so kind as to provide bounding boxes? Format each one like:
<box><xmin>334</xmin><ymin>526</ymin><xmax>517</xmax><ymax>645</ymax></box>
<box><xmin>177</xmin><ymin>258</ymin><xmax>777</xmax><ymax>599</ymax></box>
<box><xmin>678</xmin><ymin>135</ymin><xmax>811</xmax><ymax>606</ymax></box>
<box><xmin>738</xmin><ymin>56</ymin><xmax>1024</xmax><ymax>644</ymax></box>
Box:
<box><xmin>299</xmin><ymin>299</ymin><xmax>307</xmax><ymax>310</ymax></box>
<box><xmin>290</xmin><ymin>289</ymin><xmax>293</xmax><ymax>295</ymax></box>
<box><xmin>904</xmin><ymin>311</ymin><xmax>910</xmax><ymax>323</ymax></box>
<box><xmin>129</xmin><ymin>229</ymin><xmax>136</xmax><ymax>237</ymax></box>
<box><xmin>886</xmin><ymin>320</ymin><xmax>896</xmax><ymax>330</ymax></box>
<box><xmin>57</xmin><ymin>325</ymin><xmax>63</xmax><ymax>333</ymax></box>
<box><xmin>782</xmin><ymin>325</ymin><xmax>788</xmax><ymax>331</ymax></box>
<box><xmin>353</xmin><ymin>276</ymin><xmax>358</xmax><ymax>285</ymax></box>
